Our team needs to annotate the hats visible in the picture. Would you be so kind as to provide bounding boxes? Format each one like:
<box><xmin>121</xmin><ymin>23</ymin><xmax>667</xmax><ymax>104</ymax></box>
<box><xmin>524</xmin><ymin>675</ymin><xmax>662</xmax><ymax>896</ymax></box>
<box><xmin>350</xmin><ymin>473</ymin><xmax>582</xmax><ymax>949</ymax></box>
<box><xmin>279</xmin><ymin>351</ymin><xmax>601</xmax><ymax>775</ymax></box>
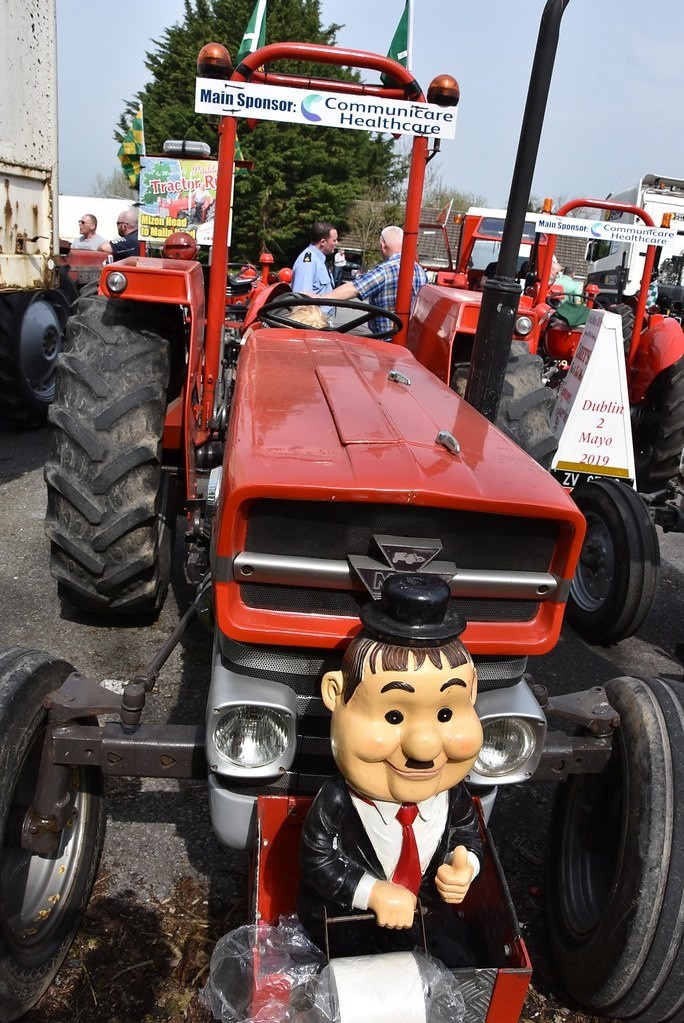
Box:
<box><xmin>563</xmin><ymin>265</ymin><xmax>576</xmax><ymax>274</ymax></box>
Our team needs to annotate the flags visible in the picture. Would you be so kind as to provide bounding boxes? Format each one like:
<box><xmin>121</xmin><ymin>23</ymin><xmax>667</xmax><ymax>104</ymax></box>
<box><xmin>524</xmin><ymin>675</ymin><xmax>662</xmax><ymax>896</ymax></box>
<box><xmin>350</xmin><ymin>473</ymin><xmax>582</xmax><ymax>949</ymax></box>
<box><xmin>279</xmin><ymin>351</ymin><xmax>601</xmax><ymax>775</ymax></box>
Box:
<box><xmin>435</xmin><ymin>198</ymin><xmax>453</xmax><ymax>223</ymax></box>
<box><xmin>380</xmin><ymin>0</ymin><xmax>413</xmax><ymax>81</ymax></box>
<box><xmin>236</xmin><ymin>0</ymin><xmax>267</xmax><ymax>66</ymax></box>
<box><xmin>118</xmin><ymin>109</ymin><xmax>145</xmax><ymax>184</ymax></box>
<box><xmin>234</xmin><ymin>134</ymin><xmax>244</xmax><ymax>171</ymax></box>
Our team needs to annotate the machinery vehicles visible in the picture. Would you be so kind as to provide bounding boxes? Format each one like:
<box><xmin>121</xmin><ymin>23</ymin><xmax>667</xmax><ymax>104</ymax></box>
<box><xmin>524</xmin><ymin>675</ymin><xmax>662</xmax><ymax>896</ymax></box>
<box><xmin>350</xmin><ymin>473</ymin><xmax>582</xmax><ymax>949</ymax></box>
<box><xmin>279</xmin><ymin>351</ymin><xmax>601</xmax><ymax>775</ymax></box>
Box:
<box><xmin>184</xmin><ymin>253</ymin><xmax>293</xmax><ymax>360</ymax></box>
<box><xmin>403</xmin><ymin>198</ymin><xmax>684</xmax><ymax>647</ymax></box>
<box><xmin>0</xmin><ymin>0</ymin><xmax>684</xmax><ymax>1023</ymax></box>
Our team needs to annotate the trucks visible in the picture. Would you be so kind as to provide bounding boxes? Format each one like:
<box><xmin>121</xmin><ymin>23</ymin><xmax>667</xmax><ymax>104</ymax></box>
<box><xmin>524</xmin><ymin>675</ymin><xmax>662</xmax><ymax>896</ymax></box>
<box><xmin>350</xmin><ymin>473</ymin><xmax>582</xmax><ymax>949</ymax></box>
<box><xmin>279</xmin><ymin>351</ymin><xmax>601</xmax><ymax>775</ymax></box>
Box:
<box><xmin>0</xmin><ymin>1</ymin><xmax>236</xmax><ymax>435</ymax></box>
<box><xmin>584</xmin><ymin>173</ymin><xmax>684</xmax><ymax>330</ymax></box>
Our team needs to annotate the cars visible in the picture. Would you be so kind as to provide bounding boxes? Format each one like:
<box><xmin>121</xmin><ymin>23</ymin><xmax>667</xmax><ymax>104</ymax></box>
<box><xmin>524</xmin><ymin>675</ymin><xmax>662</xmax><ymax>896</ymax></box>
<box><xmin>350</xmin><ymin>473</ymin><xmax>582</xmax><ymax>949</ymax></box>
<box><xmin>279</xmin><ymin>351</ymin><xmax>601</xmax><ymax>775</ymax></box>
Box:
<box><xmin>330</xmin><ymin>246</ymin><xmax>365</xmax><ymax>284</ymax></box>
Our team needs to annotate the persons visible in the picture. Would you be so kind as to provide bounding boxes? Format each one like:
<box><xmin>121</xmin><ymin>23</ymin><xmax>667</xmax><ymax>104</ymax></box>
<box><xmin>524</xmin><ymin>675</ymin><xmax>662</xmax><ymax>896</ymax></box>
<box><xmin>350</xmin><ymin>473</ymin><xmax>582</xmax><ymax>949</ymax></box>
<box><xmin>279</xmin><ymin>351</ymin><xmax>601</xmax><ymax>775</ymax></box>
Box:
<box><xmin>99</xmin><ymin>211</ymin><xmax>140</xmax><ymax>260</ymax></box>
<box><xmin>553</xmin><ymin>267</ymin><xmax>581</xmax><ymax>304</ymax></box>
<box><xmin>302</xmin><ymin>226</ymin><xmax>426</xmax><ymax>339</ymax></box>
<box><xmin>192</xmin><ymin>183</ymin><xmax>210</xmax><ymax>216</ymax></box>
<box><xmin>291</xmin><ymin>221</ymin><xmax>337</xmax><ymax>324</ymax></box>
<box><xmin>334</xmin><ymin>249</ymin><xmax>346</xmax><ymax>285</ymax></box>
<box><xmin>286</xmin><ymin>305</ymin><xmax>330</xmax><ymax>329</ymax></box>
<box><xmin>71</xmin><ymin>214</ymin><xmax>105</xmax><ymax>251</ymax></box>
<box><xmin>297</xmin><ymin>572</ymin><xmax>485</xmax><ymax>968</ymax></box>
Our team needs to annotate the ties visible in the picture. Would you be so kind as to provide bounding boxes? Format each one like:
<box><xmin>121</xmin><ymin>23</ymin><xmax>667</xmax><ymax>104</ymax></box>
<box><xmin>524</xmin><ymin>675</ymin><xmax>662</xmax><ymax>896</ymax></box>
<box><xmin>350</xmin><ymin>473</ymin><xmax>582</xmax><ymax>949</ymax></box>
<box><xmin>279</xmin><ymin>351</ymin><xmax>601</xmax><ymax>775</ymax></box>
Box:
<box><xmin>325</xmin><ymin>261</ymin><xmax>336</xmax><ymax>316</ymax></box>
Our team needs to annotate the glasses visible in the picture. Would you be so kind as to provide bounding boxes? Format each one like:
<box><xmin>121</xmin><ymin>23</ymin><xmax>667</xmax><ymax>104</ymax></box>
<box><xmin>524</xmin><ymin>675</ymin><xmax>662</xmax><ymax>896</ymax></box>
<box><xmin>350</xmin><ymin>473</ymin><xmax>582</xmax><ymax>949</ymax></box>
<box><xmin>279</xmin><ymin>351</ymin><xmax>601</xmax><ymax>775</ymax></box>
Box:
<box><xmin>78</xmin><ymin>220</ymin><xmax>85</xmax><ymax>225</ymax></box>
<box><xmin>116</xmin><ymin>222</ymin><xmax>129</xmax><ymax>226</ymax></box>
